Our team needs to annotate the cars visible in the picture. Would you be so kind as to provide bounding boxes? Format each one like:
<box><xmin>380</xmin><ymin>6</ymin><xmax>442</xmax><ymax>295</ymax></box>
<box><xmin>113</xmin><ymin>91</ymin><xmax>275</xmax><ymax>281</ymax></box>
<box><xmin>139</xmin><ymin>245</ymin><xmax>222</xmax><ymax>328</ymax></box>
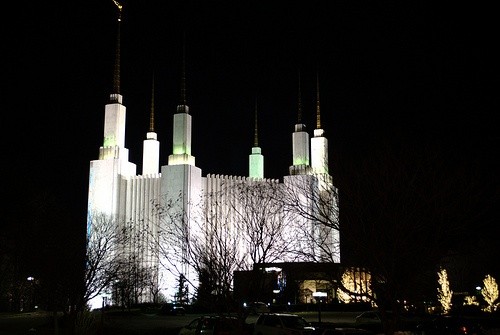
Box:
<box><xmin>425</xmin><ymin>316</ymin><xmax>483</xmax><ymax>335</ymax></box>
<box><xmin>196</xmin><ymin>315</ymin><xmax>248</xmax><ymax>335</ymax></box>
<box><xmin>356</xmin><ymin>312</ymin><xmax>402</xmax><ymax>330</ymax></box>
<box><xmin>254</xmin><ymin>313</ymin><xmax>319</xmax><ymax>335</ymax></box>
